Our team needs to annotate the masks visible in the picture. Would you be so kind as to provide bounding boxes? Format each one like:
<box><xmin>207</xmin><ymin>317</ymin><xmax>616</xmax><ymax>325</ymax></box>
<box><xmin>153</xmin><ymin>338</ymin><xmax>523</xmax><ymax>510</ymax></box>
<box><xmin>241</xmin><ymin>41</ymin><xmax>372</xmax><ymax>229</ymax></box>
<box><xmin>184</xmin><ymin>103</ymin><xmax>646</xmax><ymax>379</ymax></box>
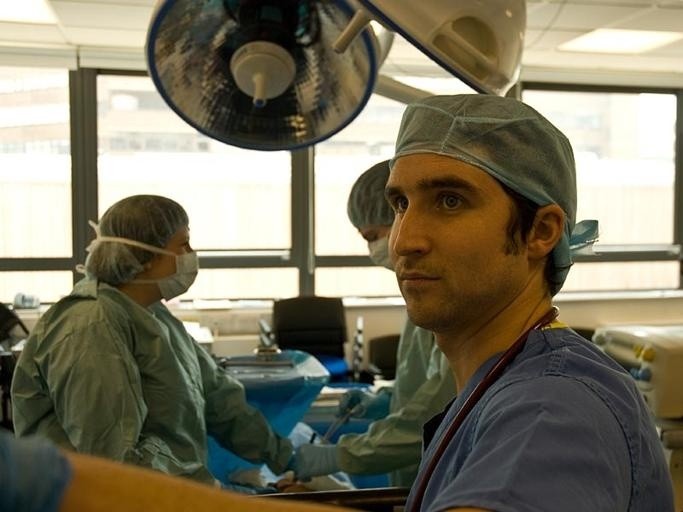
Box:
<box><xmin>366</xmin><ymin>231</ymin><xmax>394</xmax><ymax>275</ymax></box>
<box><xmin>158</xmin><ymin>251</ymin><xmax>198</xmax><ymax>302</ymax></box>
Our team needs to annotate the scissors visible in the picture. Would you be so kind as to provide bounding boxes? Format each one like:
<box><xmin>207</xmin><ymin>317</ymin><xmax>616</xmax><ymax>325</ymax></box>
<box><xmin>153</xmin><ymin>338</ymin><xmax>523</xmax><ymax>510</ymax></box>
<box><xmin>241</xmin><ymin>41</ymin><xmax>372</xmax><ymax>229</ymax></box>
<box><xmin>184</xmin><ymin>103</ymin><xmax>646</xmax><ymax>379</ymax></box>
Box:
<box><xmin>320</xmin><ymin>392</ymin><xmax>367</xmax><ymax>442</ymax></box>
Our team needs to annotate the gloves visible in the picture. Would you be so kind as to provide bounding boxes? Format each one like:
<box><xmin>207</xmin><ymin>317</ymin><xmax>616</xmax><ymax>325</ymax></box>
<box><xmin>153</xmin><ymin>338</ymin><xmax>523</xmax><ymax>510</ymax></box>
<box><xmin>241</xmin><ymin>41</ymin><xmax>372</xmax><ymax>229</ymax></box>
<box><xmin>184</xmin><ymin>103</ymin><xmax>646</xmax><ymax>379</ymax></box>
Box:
<box><xmin>333</xmin><ymin>388</ymin><xmax>389</xmax><ymax>422</ymax></box>
<box><xmin>292</xmin><ymin>444</ymin><xmax>342</xmax><ymax>482</ymax></box>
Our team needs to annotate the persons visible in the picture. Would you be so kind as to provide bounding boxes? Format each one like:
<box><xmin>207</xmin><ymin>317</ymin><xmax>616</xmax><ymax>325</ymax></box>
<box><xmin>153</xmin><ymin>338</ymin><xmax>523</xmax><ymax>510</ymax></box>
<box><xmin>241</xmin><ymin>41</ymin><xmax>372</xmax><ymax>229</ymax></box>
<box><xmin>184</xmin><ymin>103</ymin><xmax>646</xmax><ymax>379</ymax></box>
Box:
<box><xmin>0</xmin><ymin>93</ymin><xmax>680</xmax><ymax>512</ymax></box>
<box><xmin>283</xmin><ymin>157</ymin><xmax>457</xmax><ymax>479</ymax></box>
<box><xmin>6</xmin><ymin>193</ymin><xmax>294</xmax><ymax>497</ymax></box>
